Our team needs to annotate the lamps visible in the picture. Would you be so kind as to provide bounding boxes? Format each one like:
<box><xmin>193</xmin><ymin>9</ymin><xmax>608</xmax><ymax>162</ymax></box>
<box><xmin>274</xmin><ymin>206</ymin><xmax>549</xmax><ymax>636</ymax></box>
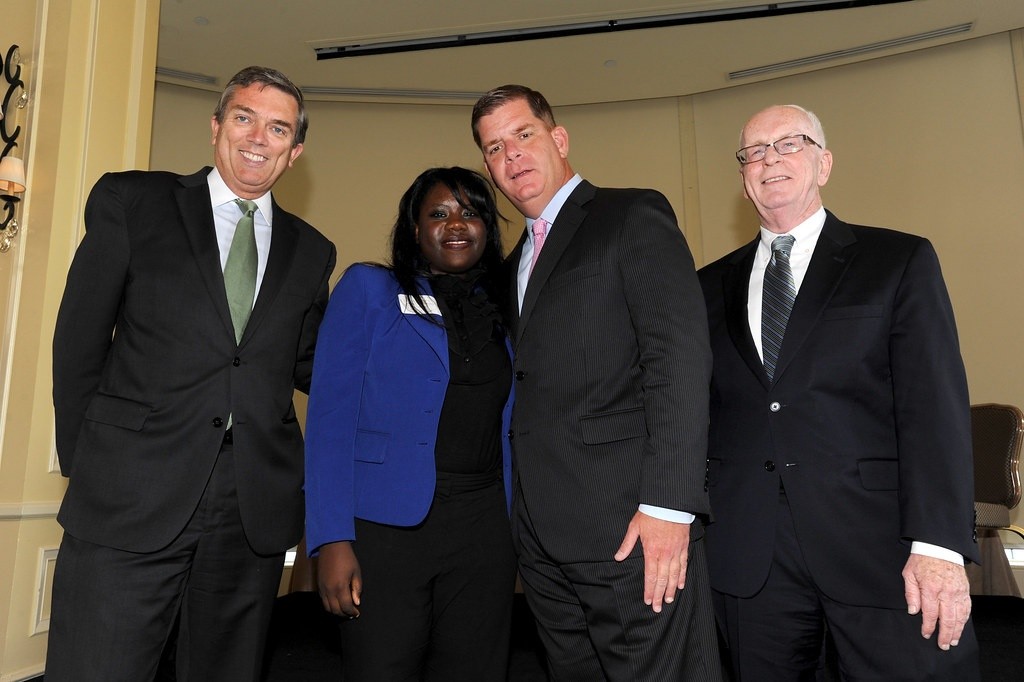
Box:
<box><xmin>0</xmin><ymin>41</ymin><xmax>28</xmax><ymax>254</ymax></box>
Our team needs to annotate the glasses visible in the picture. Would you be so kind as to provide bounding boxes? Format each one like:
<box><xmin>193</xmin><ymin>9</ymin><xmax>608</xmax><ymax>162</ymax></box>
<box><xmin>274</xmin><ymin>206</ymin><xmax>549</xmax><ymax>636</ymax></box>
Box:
<box><xmin>736</xmin><ymin>133</ymin><xmax>823</xmax><ymax>165</ymax></box>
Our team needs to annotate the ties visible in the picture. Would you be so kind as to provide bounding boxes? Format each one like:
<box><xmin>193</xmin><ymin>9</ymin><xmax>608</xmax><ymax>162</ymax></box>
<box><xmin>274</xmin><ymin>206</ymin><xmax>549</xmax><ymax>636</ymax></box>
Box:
<box><xmin>223</xmin><ymin>198</ymin><xmax>259</xmax><ymax>430</ymax></box>
<box><xmin>761</xmin><ymin>235</ymin><xmax>797</xmax><ymax>386</ymax></box>
<box><xmin>529</xmin><ymin>219</ymin><xmax>547</xmax><ymax>280</ymax></box>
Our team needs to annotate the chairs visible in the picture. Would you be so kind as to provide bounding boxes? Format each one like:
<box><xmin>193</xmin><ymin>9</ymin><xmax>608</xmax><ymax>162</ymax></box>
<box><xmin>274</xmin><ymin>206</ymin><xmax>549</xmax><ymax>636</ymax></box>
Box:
<box><xmin>972</xmin><ymin>404</ymin><xmax>1024</xmax><ymax>596</ymax></box>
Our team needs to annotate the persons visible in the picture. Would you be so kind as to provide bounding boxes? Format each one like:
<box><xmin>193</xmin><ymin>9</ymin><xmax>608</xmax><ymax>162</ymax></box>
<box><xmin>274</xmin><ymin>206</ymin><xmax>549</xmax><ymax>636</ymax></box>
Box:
<box><xmin>471</xmin><ymin>83</ymin><xmax>730</xmax><ymax>682</ymax></box>
<box><xmin>303</xmin><ymin>165</ymin><xmax>518</xmax><ymax>682</ymax></box>
<box><xmin>696</xmin><ymin>101</ymin><xmax>995</xmax><ymax>682</ymax></box>
<box><xmin>43</xmin><ymin>65</ymin><xmax>340</xmax><ymax>682</ymax></box>
